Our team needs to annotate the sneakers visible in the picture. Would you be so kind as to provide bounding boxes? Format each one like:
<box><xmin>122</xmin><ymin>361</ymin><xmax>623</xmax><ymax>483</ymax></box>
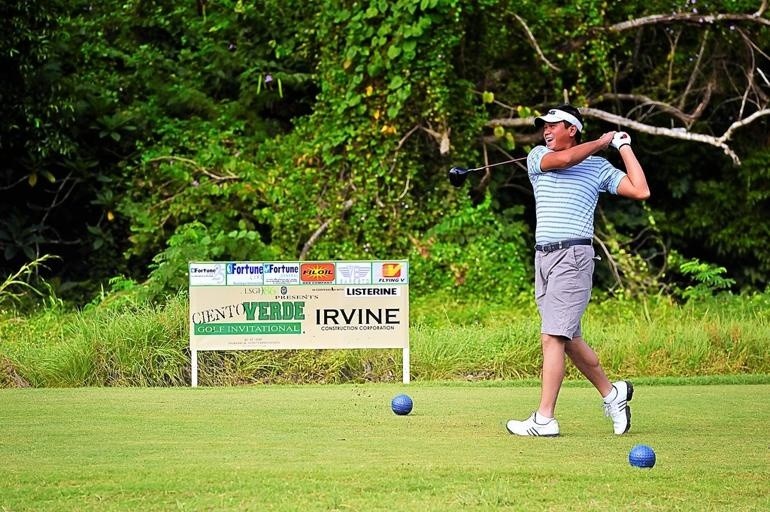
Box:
<box><xmin>506</xmin><ymin>412</ymin><xmax>560</xmax><ymax>436</ymax></box>
<box><xmin>604</xmin><ymin>381</ymin><xmax>633</xmax><ymax>434</ymax></box>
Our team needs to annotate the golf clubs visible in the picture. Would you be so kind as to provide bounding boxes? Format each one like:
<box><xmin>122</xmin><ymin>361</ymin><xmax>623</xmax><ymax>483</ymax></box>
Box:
<box><xmin>448</xmin><ymin>158</ymin><xmax>526</xmax><ymax>187</ymax></box>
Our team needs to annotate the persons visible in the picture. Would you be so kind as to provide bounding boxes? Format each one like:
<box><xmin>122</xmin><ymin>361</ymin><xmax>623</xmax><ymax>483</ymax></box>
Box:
<box><xmin>506</xmin><ymin>105</ymin><xmax>633</xmax><ymax>437</ymax></box>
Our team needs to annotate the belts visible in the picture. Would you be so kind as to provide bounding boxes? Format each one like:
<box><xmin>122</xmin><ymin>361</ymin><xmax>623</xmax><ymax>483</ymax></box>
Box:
<box><xmin>536</xmin><ymin>238</ymin><xmax>591</xmax><ymax>252</ymax></box>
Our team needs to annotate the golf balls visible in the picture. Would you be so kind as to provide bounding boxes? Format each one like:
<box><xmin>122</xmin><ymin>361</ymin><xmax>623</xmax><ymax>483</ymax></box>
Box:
<box><xmin>629</xmin><ymin>446</ymin><xmax>655</xmax><ymax>467</ymax></box>
<box><xmin>391</xmin><ymin>395</ymin><xmax>413</xmax><ymax>415</ymax></box>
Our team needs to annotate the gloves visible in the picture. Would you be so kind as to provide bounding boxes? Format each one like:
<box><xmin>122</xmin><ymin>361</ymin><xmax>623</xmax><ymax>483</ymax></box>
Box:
<box><xmin>610</xmin><ymin>131</ymin><xmax>632</xmax><ymax>150</ymax></box>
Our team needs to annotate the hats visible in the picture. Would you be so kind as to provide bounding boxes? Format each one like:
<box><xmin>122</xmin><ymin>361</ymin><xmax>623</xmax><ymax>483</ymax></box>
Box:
<box><xmin>533</xmin><ymin>109</ymin><xmax>583</xmax><ymax>133</ymax></box>
